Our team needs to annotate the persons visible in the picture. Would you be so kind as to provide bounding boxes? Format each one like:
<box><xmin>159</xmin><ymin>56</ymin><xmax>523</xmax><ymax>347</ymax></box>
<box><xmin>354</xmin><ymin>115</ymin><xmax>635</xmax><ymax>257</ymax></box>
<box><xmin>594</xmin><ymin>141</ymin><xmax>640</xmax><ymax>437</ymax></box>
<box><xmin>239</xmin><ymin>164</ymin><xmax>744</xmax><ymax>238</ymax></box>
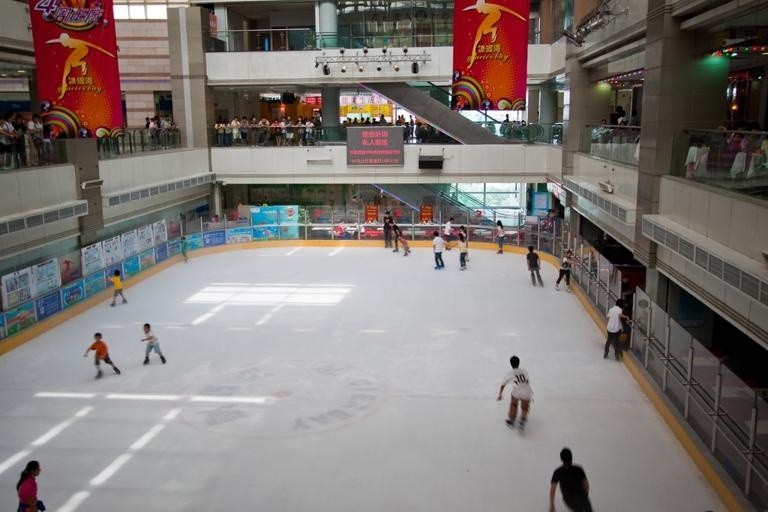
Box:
<box><xmin>105</xmin><ymin>267</ymin><xmax>127</xmax><ymax>306</ymax></box>
<box><xmin>14</xmin><ymin>459</ymin><xmax>45</xmax><ymax>512</ymax></box>
<box><xmin>2</xmin><ymin>98</ymin><xmax>53</xmax><ymax>167</ymax></box>
<box><xmin>730</xmin><ymin>122</ymin><xmax>763</xmax><ymax>153</ymax></box>
<box><xmin>498</xmin><ymin>355</ymin><xmax>534</xmax><ymax>433</ymax></box>
<box><xmin>505</xmin><ymin>114</ymin><xmax>510</xmax><ymax>122</ymax></box>
<box><xmin>145</xmin><ymin>116</ymin><xmax>176</xmax><ymax>149</ymax></box>
<box><xmin>603</xmin><ymin>298</ymin><xmax>630</xmax><ymax>359</ymax></box>
<box><xmin>593</xmin><ymin>104</ymin><xmax>638</xmax><ymax>144</ymax></box>
<box><xmin>177</xmin><ymin>237</ymin><xmax>190</xmax><ymax>263</ymax></box>
<box><xmin>81</xmin><ymin>332</ymin><xmax>121</xmax><ymax>379</ymax></box>
<box><xmin>549</xmin><ymin>446</ymin><xmax>593</xmax><ymax>510</ymax></box>
<box><xmin>374</xmin><ymin>210</ymin><xmax>576</xmax><ymax>294</ymax></box>
<box><xmin>214</xmin><ymin>115</ymin><xmax>429</xmax><ymax>145</ymax></box>
<box><xmin>141</xmin><ymin>323</ymin><xmax>168</xmax><ymax>366</ymax></box>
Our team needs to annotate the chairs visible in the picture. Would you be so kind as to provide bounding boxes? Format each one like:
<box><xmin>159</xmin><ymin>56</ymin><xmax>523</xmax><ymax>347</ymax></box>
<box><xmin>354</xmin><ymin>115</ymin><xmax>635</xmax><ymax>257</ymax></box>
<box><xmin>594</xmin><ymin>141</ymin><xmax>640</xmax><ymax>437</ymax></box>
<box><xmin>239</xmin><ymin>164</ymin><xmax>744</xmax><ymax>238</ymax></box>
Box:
<box><xmin>590</xmin><ymin>125</ymin><xmax>768</xmax><ymax>179</ymax></box>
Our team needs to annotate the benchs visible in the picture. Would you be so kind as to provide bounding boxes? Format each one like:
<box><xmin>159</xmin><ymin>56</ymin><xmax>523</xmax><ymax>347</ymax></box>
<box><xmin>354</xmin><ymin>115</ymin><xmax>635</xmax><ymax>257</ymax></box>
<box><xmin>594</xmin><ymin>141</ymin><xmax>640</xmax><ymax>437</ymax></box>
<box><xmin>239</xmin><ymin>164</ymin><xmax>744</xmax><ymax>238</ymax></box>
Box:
<box><xmin>312</xmin><ymin>221</ymin><xmax>523</xmax><ymax>244</ymax></box>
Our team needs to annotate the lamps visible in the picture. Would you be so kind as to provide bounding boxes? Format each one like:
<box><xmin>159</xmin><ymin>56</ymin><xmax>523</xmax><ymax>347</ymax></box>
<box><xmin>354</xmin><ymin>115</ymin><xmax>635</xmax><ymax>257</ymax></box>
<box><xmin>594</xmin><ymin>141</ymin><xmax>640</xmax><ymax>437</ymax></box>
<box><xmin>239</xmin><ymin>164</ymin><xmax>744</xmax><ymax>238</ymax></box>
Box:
<box><xmin>305</xmin><ymin>42</ymin><xmax>431</xmax><ymax>77</ymax></box>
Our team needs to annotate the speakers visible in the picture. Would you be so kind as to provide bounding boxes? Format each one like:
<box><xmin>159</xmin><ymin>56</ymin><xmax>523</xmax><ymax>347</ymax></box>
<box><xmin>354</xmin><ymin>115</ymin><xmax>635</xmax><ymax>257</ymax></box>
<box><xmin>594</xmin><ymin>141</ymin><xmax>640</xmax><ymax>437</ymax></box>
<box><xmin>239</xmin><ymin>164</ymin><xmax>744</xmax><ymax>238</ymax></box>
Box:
<box><xmin>418</xmin><ymin>156</ymin><xmax>443</xmax><ymax>170</ymax></box>
